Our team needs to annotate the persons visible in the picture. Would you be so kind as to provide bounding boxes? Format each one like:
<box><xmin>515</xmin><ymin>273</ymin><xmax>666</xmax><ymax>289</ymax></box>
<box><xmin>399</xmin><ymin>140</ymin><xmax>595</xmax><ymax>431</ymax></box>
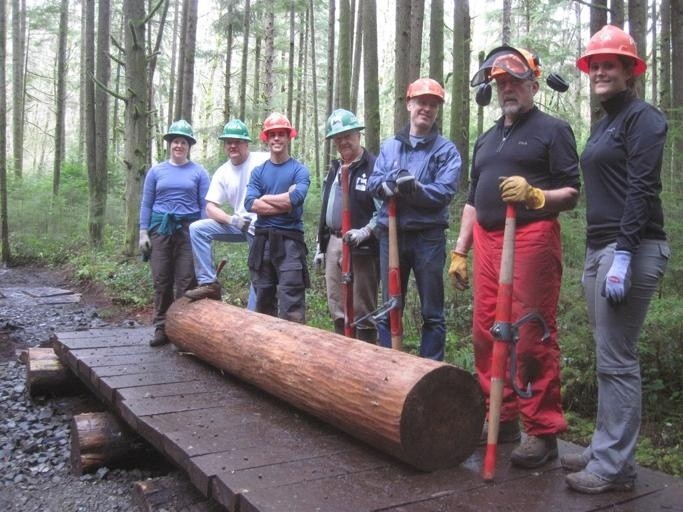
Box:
<box><xmin>312</xmin><ymin>108</ymin><xmax>381</xmax><ymax>343</ymax></box>
<box><xmin>184</xmin><ymin>117</ymin><xmax>270</xmax><ymax>312</ymax></box>
<box><xmin>559</xmin><ymin>24</ymin><xmax>670</xmax><ymax>492</ymax></box>
<box><xmin>365</xmin><ymin>76</ymin><xmax>463</xmax><ymax>363</ymax></box>
<box><xmin>447</xmin><ymin>45</ymin><xmax>582</xmax><ymax>471</ymax></box>
<box><xmin>242</xmin><ymin>111</ymin><xmax>310</xmax><ymax>322</ymax></box>
<box><xmin>138</xmin><ymin>118</ymin><xmax>209</xmax><ymax>346</ymax></box>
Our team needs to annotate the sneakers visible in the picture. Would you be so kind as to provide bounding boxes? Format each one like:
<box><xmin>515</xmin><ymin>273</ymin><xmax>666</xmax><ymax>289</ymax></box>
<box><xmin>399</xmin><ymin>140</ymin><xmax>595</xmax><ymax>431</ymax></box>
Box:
<box><xmin>477</xmin><ymin>416</ymin><xmax>521</xmax><ymax>444</ymax></box>
<box><xmin>183</xmin><ymin>281</ymin><xmax>221</xmax><ymax>299</ymax></box>
<box><xmin>560</xmin><ymin>444</ymin><xmax>593</xmax><ymax>472</ymax></box>
<box><xmin>564</xmin><ymin>468</ymin><xmax>635</xmax><ymax>494</ymax></box>
<box><xmin>509</xmin><ymin>433</ymin><xmax>558</xmax><ymax>467</ymax></box>
<box><xmin>149</xmin><ymin>328</ymin><xmax>168</xmax><ymax>347</ymax></box>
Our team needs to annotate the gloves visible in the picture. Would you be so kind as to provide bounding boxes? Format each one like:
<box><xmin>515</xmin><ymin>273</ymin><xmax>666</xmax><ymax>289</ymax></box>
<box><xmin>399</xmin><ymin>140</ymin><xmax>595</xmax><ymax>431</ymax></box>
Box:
<box><xmin>138</xmin><ymin>229</ymin><xmax>153</xmax><ymax>257</ymax></box>
<box><xmin>313</xmin><ymin>242</ymin><xmax>325</xmax><ymax>274</ymax></box>
<box><xmin>341</xmin><ymin>227</ymin><xmax>370</xmax><ymax>249</ymax></box>
<box><xmin>497</xmin><ymin>176</ymin><xmax>545</xmax><ymax>212</ymax></box>
<box><xmin>600</xmin><ymin>250</ymin><xmax>633</xmax><ymax>305</ymax></box>
<box><xmin>228</xmin><ymin>215</ymin><xmax>251</xmax><ymax>232</ymax></box>
<box><xmin>375</xmin><ymin>169</ymin><xmax>419</xmax><ymax>202</ymax></box>
<box><xmin>447</xmin><ymin>251</ymin><xmax>470</xmax><ymax>292</ymax></box>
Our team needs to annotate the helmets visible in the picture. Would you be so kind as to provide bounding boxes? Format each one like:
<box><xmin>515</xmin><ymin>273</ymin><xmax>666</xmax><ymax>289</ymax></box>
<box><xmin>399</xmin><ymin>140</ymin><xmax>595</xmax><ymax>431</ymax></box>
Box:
<box><xmin>406</xmin><ymin>78</ymin><xmax>445</xmax><ymax>103</ymax></box>
<box><xmin>470</xmin><ymin>44</ymin><xmax>542</xmax><ymax>87</ymax></box>
<box><xmin>162</xmin><ymin>119</ymin><xmax>196</xmax><ymax>144</ymax></box>
<box><xmin>576</xmin><ymin>25</ymin><xmax>646</xmax><ymax>77</ymax></box>
<box><xmin>218</xmin><ymin>119</ymin><xmax>252</xmax><ymax>143</ymax></box>
<box><xmin>324</xmin><ymin>109</ymin><xmax>366</xmax><ymax>140</ymax></box>
<box><xmin>260</xmin><ymin>112</ymin><xmax>297</xmax><ymax>142</ymax></box>
